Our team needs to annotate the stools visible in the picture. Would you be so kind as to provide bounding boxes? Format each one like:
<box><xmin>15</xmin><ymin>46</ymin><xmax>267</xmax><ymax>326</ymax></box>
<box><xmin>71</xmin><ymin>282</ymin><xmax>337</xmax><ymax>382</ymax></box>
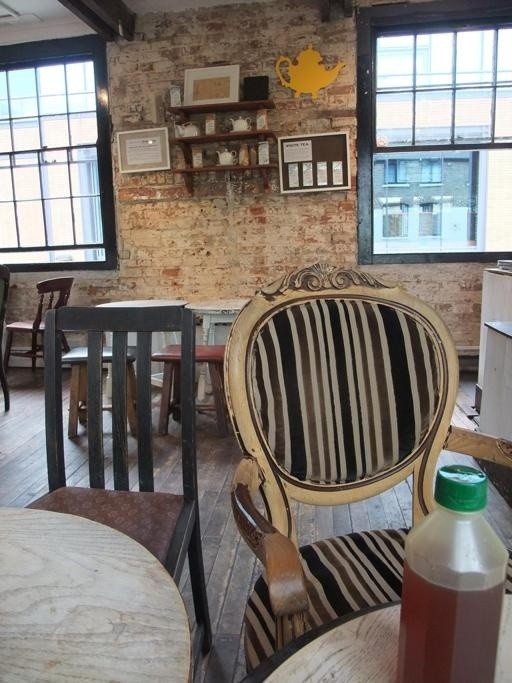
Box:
<box><xmin>61</xmin><ymin>346</ymin><xmax>136</xmax><ymax>437</ymax></box>
<box><xmin>151</xmin><ymin>345</ymin><xmax>226</xmax><ymax>437</ymax></box>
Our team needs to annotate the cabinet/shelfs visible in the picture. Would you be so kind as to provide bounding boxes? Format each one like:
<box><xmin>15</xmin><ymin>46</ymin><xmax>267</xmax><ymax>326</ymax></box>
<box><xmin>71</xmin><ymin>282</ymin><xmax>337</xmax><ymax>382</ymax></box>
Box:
<box><xmin>478</xmin><ymin>322</ymin><xmax>512</xmax><ymax>444</ymax></box>
<box><xmin>167</xmin><ymin>99</ymin><xmax>279</xmax><ymax>193</ymax></box>
<box><xmin>476</xmin><ymin>268</ymin><xmax>512</xmax><ymax>414</ymax></box>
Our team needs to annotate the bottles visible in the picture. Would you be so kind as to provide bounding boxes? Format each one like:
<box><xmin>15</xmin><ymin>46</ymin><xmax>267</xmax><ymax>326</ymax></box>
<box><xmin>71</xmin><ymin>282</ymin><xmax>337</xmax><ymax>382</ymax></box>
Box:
<box><xmin>398</xmin><ymin>464</ymin><xmax>510</xmax><ymax>683</ymax></box>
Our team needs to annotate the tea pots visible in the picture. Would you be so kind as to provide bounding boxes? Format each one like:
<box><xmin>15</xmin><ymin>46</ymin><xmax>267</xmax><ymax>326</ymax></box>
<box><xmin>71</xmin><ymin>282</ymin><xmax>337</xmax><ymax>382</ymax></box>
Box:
<box><xmin>178</xmin><ymin>120</ymin><xmax>200</xmax><ymax>138</ymax></box>
<box><xmin>229</xmin><ymin>116</ymin><xmax>251</xmax><ymax>131</ymax></box>
<box><xmin>215</xmin><ymin>148</ymin><xmax>237</xmax><ymax>165</ymax></box>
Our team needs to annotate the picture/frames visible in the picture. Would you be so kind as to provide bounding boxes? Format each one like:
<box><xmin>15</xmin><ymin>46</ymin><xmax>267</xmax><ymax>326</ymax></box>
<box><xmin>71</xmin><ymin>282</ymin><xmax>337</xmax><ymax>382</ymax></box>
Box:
<box><xmin>116</xmin><ymin>126</ymin><xmax>171</xmax><ymax>173</ymax></box>
<box><xmin>243</xmin><ymin>76</ymin><xmax>269</xmax><ymax>101</ymax></box>
<box><xmin>184</xmin><ymin>64</ymin><xmax>239</xmax><ymax>105</ymax></box>
<box><xmin>277</xmin><ymin>131</ymin><xmax>351</xmax><ymax>194</ymax></box>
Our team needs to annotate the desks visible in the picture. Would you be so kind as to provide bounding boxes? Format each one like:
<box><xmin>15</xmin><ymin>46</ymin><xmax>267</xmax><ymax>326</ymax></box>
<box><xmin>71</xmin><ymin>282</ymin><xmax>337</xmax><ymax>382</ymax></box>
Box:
<box><xmin>185</xmin><ymin>299</ymin><xmax>251</xmax><ymax>402</ymax></box>
<box><xmin>95</xmin><ymin>300</ymin><xmax>189</xmax><ymax>398</ymax></box>
<box><xmin>0</xmin><ymin>508</ymin><xmax>195</xmax><ymax>683</ymax></box>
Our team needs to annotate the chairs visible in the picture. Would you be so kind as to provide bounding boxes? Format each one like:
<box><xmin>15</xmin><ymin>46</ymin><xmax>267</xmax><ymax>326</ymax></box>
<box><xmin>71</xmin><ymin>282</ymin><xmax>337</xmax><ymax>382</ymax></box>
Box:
<box><xmin>22</xmin><ymin>305</ymin><xmax>213</xmax><ymax>683</ymax></box>
<box><xmin>0</xmin><ymin>264</ymin><xmax>10</xmax><ymax>412</ymax></box>
<box><xmin>3</xmin><ymin>277</ymin><xmax>75</xmax><ymax>376</ymax></box>
<box><xmin>223</xmin><ymin>261</ymin><xmax>512</xmax><ymax>678</ymax></box>
